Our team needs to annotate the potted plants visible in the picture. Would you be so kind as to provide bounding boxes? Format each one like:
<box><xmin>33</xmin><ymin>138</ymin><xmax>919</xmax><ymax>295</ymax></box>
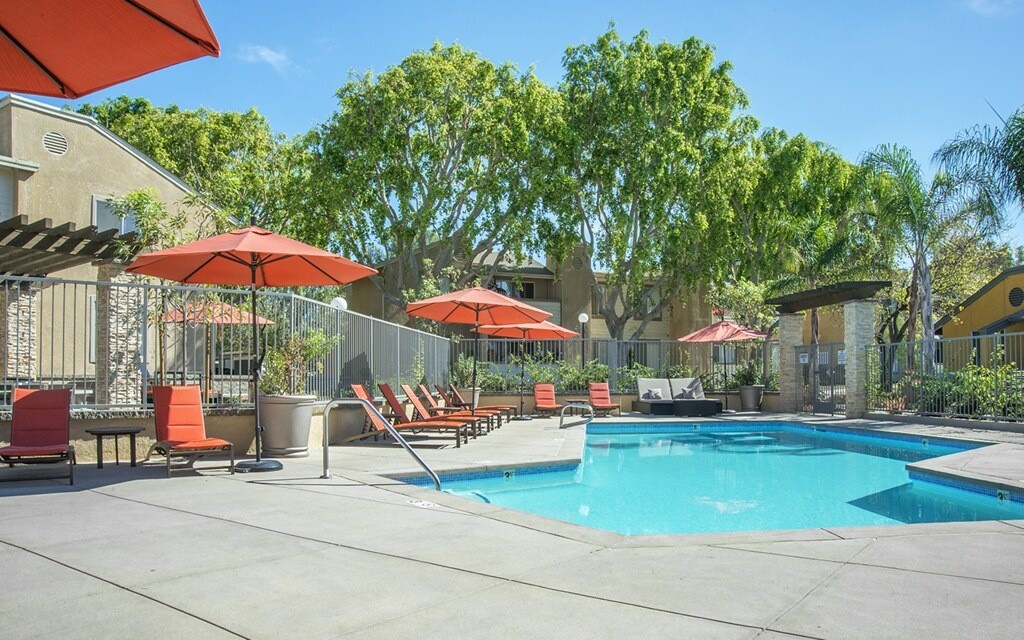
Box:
<box><xmin>731</xmin><ymin>356</ymin><xmax>765</xmax><ymax>412</ymax></box>
<box><xmin>248</xmin><ymin>327</ymin><xmax>346</xmax><ymax>459</ymax></box>
<box><xmin>454</xmin><ymin>351</ymin><xmax>481</xmax><ymax>409</ymax></box>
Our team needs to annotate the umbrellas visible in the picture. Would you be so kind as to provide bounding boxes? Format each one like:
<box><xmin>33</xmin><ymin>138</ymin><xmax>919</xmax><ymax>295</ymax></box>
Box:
<box><xmin>141</xmin><ymin>294</ymin><xmax>275</xmax><ymax>404</ymax></box>
<box><xmin>127</xmin><ymin>217</ymin><xmax>379</xmax><ymax>461</ymax></box>
<box><xmin>0</xmin><ymin>0</ymin><xmax>221</xmax><ymax>100</ymax></box>
<box><xmin>471</xmin><ymin>320</ymin><xmax>581</xmax><ymax>417</ymax></box>
<box><xmin>677</xmin><ymin>321</ymin><xmax>768</xmax><ymax>412</ymax></box>
<box><xmin>406</xmin><ymin>286</ymin><xmax>553</xmax><ymax>430</ymax></box>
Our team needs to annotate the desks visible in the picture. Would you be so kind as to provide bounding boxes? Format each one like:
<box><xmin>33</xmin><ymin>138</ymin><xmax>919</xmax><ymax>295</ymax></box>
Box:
<box><xmin>429</xmin><ymin>406</ymin><xmax>462</xmax><ymax>417</ymax></box>
<box><xmin>85</xmin><ymin>427</ymin><xmax>146</xmax><ymax>469</ymax></box>
<box><xmin>448</xmin><ymin>403</ymin><xmax>477</xmax><ymax>411</ymax></box>
<box><xmin>371</xmin><ymin>413</ymin><xmax>404</xmax><ymax>440</ymax></box>
<box><xmin>566</xmin><ymin>399</ymin><xmax>588</xmax><ymax>417</ymax></box>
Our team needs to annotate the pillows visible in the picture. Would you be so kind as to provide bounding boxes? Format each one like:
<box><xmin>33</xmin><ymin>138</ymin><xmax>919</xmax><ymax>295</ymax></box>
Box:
<box><xmin>681</xmin><ymin>388</ymin><xmax>696</xmax><ymax>399</ymax></box>
<box><xmin>647</xmin><ymin>388</ymin><xmax>663</xmax><ymax>399</ymax></box>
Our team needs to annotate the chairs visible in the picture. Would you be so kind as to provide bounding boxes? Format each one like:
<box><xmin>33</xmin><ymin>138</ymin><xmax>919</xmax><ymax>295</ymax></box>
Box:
<box><xmin>351</xmin><ymin>384</ymin><xmax>468</xmax><ymax>449</ymax></box>
<box><xmin>434</xmin><ymin>384</ymin><xmax>511</xmax><ymax>423</ymax></box>
<box><xmin>400</xmin><ymin>385</ymin><xmax>495</xmax><ymax>432</ymax></box>
<box><xmin>531</xmin><ymin>383</ymin><xmax>563</xmax><ymax>416</ymax></box>
<box><xmin>146</xmin><ymin>389</ymin><xmax>220</xmax><ymax>404</ymax></box>
<box><xmin>631</xmin><ymin>378</ymin><xmax>676</xmax><ymax>415</ymax></box>
<box><xmin>0</xmin><ymin>387</ymin><xmax>76</xmax><ymax>486</ymax></box>
<box><xmin>669</xmin><ymin>378</ymin><xmax>723</xmax><ymax>414</ymax></box>
<box><xmin>588</xmin><ymin>383</ymin><xmax>621</xmax><ymax>417</ymax></box>
<box><xmin>378</xmin><ymin>384</ymin><xmax>482</xmax><ymax>439</ymax></box>
<box><xmin>145</xmin><ymin>386</ymin><xmax>236</xmax><ymax>478</ymax></box>
<box><xmin>419</xmin><ymin>385</ymin><xmax>503</xmax><ymax>428</ymax></box>
<box><xmin>449</xmin><ymin>385</ymin><xmax>518</xmax><ymax>417</ymax></box>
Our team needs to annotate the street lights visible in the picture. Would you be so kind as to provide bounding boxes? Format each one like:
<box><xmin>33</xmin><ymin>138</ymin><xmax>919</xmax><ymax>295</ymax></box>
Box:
<box><xmin>330</xmin><ymin>296</ymin><xmax>349</xmax><ymax>399</ymax></box>
<box><xmin>578</xmin><ymin>312</ymin><xmax>590</xmax><ymax>371</ymax></box>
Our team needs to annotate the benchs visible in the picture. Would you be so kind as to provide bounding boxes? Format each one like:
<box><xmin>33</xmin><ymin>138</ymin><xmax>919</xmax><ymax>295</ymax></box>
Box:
<box><xmin>673</xmin><ymin>399</ymin><xmax>716</xmax><ymax>417</ymax></box>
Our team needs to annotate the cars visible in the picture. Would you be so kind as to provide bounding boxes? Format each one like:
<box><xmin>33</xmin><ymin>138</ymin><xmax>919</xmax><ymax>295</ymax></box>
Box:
<box><xmin>892</xmin><ymin>367</ymin><xmax>1024</xmax><ymax>424</ymax></box>
